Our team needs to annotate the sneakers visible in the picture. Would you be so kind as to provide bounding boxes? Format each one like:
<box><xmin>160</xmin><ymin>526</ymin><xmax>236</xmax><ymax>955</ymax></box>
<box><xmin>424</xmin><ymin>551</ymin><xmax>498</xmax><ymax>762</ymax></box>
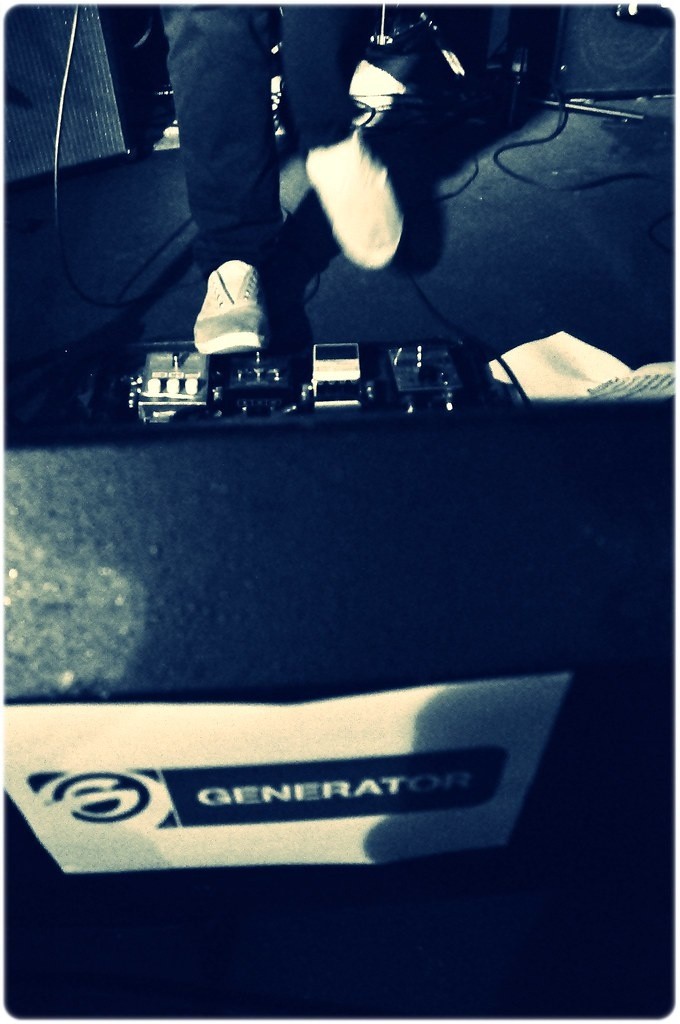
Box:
<box><xmin>305</xmin><ymin>129</ymin><xmax>405</xmax><ymax>273</ymax></box>
<box><xmin>192</xmin><ymin>264</ymin><xmax>265</xmax><ymax>356</ymax></box>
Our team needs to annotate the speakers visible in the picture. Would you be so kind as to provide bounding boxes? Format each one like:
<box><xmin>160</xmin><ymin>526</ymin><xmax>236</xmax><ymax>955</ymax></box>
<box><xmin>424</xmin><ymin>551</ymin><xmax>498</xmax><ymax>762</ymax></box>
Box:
<box><xmin>4</xmin><ymin>6</ymin><xmax>137</xmax><ymax>187</ymax></box>
<box><xmin>508</xmin><ymin>4</ymin><xmax>676</xmax><ymax>102</ymax></box>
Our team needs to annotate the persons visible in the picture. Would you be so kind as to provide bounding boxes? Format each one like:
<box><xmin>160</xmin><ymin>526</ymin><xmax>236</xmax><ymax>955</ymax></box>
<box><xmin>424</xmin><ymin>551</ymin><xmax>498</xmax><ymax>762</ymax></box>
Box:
<box><xmin>160</xmin><ymin>4</ymin><xmax>403</xmax><ymax>356</ymax></box>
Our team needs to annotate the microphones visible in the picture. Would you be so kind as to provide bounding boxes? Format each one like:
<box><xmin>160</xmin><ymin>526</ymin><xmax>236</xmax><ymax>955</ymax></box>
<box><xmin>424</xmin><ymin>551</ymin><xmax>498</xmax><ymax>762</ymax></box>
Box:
<box><xmin>612</xmin><ymin>4</ymin><xmax>664</xmax><ymax>17</ymax></box>
